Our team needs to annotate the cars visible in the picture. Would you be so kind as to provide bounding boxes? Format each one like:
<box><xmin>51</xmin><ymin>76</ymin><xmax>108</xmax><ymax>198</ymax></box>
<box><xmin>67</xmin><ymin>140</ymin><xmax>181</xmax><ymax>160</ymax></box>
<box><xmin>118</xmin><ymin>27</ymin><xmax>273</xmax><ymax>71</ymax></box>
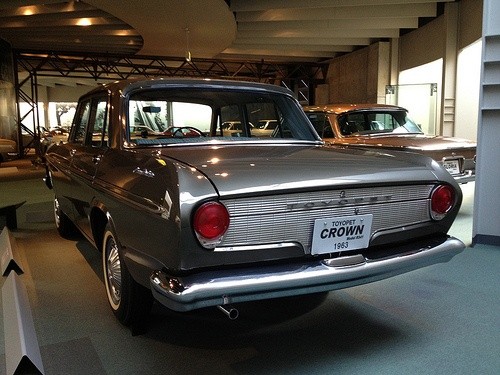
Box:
<box><xmin>44</xmin><ymin>76</ymin><xmax>477</xmax><ymax>323</ymax></box>
